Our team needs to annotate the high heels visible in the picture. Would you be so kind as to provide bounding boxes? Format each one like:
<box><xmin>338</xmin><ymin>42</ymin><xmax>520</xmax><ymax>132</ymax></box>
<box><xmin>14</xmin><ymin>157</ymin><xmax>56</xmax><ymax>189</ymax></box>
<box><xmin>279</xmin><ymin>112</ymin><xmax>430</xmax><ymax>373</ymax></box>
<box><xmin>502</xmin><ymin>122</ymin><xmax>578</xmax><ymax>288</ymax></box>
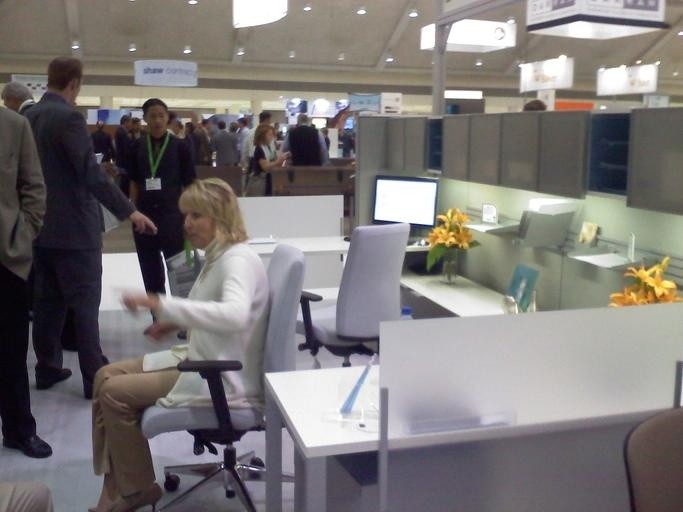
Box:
<box><xmin>108</xmin><ymin>483</ymin><xmax>162</xmax><ymax>512</ymax></box>
<box><xmin>87</xmin><ymin>494</ymin><xmax>122</xmax><ymax>512</ymax></box>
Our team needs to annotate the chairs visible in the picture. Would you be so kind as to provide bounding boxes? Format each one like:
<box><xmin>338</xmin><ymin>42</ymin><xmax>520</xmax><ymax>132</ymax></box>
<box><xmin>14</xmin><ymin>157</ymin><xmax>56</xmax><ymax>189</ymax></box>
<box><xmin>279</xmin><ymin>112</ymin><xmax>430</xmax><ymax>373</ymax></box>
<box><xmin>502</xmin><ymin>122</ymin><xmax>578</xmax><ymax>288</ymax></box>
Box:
<box><xmin>623</xmin><ymin>401</ymin><xmax>681</xmax><ymax>512</ymax></box>
<box><xmin>297</xmin><ymin>219</ymin><xmax>412</xmax><ymax>368</ymax></box>
<box><xmin>139</xmin><ymin>240</ymin><xmax>304</xmax><ymax>510</ymax></box>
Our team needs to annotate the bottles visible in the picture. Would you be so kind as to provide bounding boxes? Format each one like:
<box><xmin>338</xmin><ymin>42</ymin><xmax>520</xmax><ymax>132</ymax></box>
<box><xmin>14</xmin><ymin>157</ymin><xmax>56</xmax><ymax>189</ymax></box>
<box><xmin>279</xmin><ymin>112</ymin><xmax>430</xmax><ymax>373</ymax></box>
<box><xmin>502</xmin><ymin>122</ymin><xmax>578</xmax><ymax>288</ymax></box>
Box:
<box><xmin>399</xmin><ymin>307</ymin><xmax>413</xmax><ymax>320</ymax></box>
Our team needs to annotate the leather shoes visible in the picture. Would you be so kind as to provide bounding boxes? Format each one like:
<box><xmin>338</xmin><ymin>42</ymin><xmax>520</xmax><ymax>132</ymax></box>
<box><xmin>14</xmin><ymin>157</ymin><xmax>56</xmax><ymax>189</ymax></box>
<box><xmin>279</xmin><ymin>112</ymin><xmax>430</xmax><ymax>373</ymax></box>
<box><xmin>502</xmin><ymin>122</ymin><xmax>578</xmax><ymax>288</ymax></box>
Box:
<box><xmin>3</xmin><ymin>434</ymin><xmax>53</xmax><ymax>459</ymax></box>
<box><xmin>35</xmin><ymin>368</ymin><xmax>73</xmax><ymax>391</ymax></box>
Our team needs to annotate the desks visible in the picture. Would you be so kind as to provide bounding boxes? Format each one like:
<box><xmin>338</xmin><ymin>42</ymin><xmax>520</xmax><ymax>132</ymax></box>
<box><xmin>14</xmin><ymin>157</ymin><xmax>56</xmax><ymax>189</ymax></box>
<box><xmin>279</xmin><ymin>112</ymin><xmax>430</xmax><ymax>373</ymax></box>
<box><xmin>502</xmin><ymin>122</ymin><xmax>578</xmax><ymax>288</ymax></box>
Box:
<box><xmin>401</xmin><ymin>269</ymin><xmax>539</xmax><ymax>322</ymax></box>
<box><xmin>190</xmin><ymin>237</ymin><xmax>351</xmax><ymax>291</ymax></box>
<box><xmin>263</xmin><ymin>304</ymin><xmax>682</xmax><ymax>512</ymax></box>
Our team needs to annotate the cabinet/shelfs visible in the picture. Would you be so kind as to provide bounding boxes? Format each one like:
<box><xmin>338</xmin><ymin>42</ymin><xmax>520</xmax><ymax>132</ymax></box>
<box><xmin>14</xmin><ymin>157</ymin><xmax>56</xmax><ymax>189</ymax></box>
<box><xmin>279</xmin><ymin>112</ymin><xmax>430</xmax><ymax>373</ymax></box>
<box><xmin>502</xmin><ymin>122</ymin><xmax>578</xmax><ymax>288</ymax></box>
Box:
<box><xmin>385</xmin><ymin>117</ymin><xmax>427</xmax><ymax>176</ymax></box>
<box><xmin>500</xmin><ymin>109</ymin><xmax>591</xmax><ymax>204</ymax></box>
<box><xmin>584</xmin><ymin>113</ymin><xmax>630</xmax><ymax>196</ymax></box>
<box><xmin>426</xmin><ymin>117</ymin><xmax>442</xmax><ymax>174</ymax></box>
<box><xmin>442</xmin><ymin>112</ymin><xmax>500</xmax><ymax>187</ymax></box>
<box><xmin>623</xmin><ymin>107</ymin><xmax>682</xmax><ymax>216</ymax></box>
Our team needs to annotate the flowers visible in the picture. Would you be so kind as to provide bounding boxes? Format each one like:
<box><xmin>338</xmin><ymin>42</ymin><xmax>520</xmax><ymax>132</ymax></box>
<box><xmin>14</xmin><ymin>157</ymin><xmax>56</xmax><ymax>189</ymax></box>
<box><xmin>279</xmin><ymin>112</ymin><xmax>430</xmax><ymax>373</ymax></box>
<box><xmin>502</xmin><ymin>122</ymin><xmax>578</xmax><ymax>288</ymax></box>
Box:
<box><xmin>423</xmin><ymin>209</ymin><xmax>475</xmax><ymax>270</ymax></box>
<box><xmin>604</xmin><ymin>256</ymin><xmax>683</xmax><ymax>308</ymax></box>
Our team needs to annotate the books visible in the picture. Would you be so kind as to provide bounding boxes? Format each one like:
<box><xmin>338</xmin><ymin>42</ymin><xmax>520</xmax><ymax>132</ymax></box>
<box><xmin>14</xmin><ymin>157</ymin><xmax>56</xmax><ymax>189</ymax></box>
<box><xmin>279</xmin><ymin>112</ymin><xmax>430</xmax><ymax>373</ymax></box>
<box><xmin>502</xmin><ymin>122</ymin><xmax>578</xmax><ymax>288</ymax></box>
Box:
<box><xmin>484</xmin><ymin>208</ymin><xmax>539</xmax><ymax>238</ymax></box>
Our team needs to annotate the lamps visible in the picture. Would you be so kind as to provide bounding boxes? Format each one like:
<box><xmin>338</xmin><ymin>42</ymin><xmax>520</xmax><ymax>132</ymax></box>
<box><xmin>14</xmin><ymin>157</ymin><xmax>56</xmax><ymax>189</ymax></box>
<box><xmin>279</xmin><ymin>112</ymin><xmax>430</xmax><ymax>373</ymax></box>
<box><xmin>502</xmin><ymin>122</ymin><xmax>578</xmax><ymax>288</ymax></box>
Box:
<box><xmin>231</xmin><ymin>0</ymin><xmax>287</xmax><ymax>30</ymax></box>
<box><xmin>420</xmin><ymin>17</ymin><xmax>518</xmax><ymax>54</ymax></box>
<box><xmin>526</xmin><ymin>0</ymin><xmax>672</xmax><ymax>42</ymax></box>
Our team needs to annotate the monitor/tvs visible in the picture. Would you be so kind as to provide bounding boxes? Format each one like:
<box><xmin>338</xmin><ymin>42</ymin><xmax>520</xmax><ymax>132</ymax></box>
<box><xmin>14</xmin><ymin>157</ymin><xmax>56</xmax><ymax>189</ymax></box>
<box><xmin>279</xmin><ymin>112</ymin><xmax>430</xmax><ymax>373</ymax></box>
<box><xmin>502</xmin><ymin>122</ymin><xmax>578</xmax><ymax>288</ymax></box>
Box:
<box><xmin>372</xmin><ymin>175</ymin><xmax>439</xmax><ymax>236</ymax></box>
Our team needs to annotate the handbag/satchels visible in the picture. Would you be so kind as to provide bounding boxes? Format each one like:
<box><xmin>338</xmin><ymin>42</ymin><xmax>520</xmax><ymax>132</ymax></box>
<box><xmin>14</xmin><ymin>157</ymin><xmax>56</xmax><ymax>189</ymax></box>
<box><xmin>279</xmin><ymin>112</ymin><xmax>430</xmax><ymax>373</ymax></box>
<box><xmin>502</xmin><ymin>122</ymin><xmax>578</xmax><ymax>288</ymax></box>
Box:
<box><xmin>167</xmin><ymin>255</ymin><xmax>208</xmax><ymax>299</ymax></box>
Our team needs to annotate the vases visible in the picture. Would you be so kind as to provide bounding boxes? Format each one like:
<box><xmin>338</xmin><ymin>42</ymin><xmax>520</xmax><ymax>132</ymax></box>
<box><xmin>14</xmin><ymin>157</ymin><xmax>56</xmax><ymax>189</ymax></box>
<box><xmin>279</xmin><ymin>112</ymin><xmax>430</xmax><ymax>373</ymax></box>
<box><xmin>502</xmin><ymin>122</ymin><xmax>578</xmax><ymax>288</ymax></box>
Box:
<box><xmin>441</xmin><ymin>257</ymin><xmax>456</xmax><ymax>283</ymax></box>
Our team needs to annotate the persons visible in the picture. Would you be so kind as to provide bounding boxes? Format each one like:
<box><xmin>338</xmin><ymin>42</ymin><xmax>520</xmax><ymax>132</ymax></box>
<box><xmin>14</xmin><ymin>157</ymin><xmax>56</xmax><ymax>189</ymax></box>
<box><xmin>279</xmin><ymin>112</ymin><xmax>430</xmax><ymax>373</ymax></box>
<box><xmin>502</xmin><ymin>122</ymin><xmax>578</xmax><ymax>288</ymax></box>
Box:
<box><xmin>19</xmin><ymin>57</ymin><xmax>162</xmax><ymax>401</ymax></box>
<box><xmin>91</xmin><ymin>176</ymin><xmax>271</xmax><ymax>511</ymax></box>
<box><xmin>89</xmin><ymin>84</ymin><xmax>357</xmax><ymax>202</ymax></box>
<box><xmin>0</xmin><ymin>105</ymin><xmax>53</xmax><ymax>459</ymax></box>
<box><xmin>115</xmin><ymin>98</ymin><xmax>198</xmax><ymax>340</ymax></box>
<box><xmin>0</xmin><ymin>82</ymin><xmax>38</xmax><ymax>116</ymax></box>
